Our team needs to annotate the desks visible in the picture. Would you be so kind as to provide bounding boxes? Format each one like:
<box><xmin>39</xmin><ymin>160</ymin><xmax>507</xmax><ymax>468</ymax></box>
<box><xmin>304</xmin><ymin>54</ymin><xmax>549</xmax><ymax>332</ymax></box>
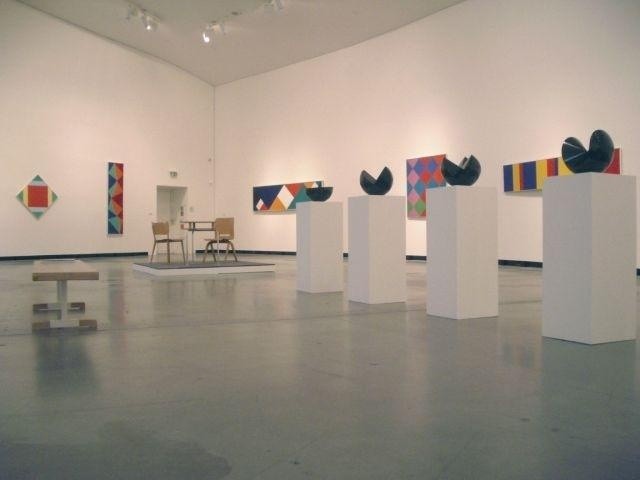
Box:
<box><xmin>179</xmin><ymin>219</ymin><xmax>219</xmax><ymax>261</ymax></box>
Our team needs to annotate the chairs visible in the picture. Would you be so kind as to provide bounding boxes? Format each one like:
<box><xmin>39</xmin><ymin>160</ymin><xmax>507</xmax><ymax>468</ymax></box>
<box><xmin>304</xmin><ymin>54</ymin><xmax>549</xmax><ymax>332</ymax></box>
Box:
<box><xmin>151</xmin><ymin>221</ymin><xmax>185</xmax><ymax>262</ymax></box>
<box><xmin>203</xmin><ymin>217</ymin><xmax>238</xmax><ymax>262</ymax></box>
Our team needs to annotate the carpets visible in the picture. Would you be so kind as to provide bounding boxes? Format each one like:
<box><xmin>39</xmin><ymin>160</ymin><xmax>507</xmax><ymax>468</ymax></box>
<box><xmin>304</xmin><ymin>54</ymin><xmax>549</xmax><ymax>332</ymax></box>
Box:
<box><xmin>132</xmin><ymin>260</ymin><xmax>274</xmax><ymax>269</ymax></box>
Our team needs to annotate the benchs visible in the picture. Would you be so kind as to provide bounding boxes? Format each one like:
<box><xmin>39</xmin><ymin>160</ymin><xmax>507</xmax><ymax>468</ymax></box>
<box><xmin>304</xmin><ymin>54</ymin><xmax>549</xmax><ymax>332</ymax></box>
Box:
<box><xmin>31</xmin><ymin>258</ymin><xmax>98</xmax><ymax>330</ymax></box>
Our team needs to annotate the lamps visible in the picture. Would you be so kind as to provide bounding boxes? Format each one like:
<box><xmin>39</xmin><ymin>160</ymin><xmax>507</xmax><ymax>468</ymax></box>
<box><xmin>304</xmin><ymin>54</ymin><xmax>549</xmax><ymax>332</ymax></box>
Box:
<box><xmin>124</xmin><ymin>0</ymin><xmax>161</xmax><ymax>31</ymax></box>
